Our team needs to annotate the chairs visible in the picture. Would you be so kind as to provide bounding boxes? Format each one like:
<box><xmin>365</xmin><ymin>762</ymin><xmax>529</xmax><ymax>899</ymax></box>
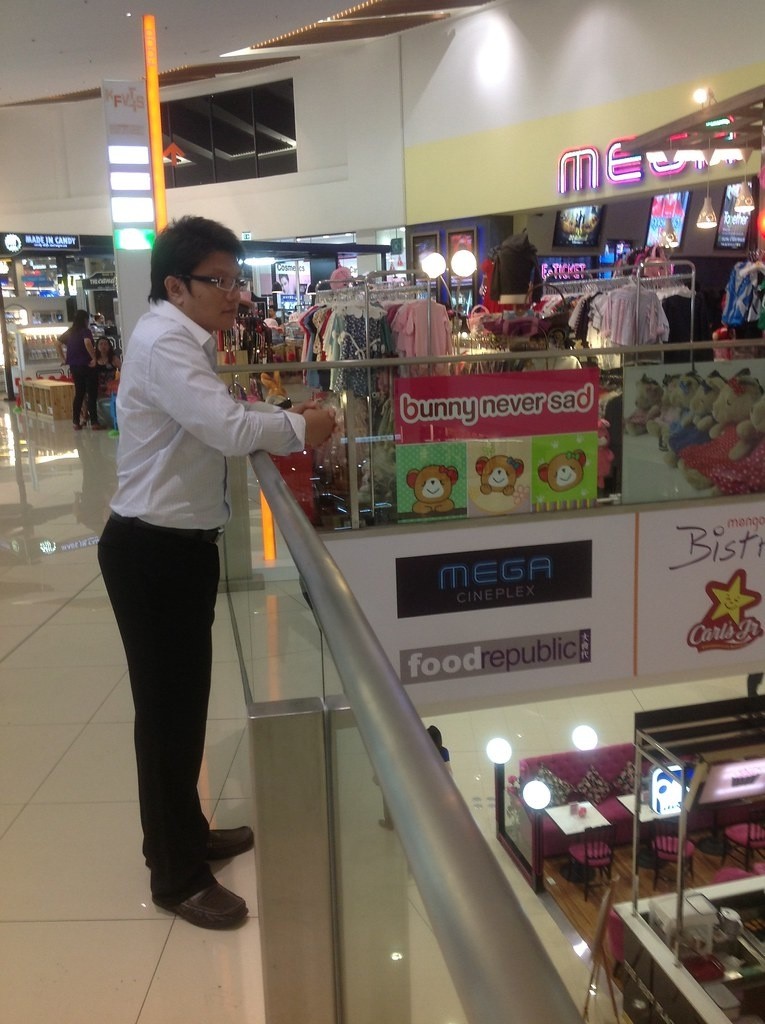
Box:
<box><xmin>568</xmin><ymin>824</ymin><xmax>616</xmax><ymax>901</ymax></box>
<box><xmin>714</xmin><ymin>863</ymin><xmax>765</xmax><ymax>884</ymax></box>
<box><xmin>652</xmin><ymin>818</ymin><xmax>695</xmax><ymax>890</ymax></box>
<box><xmin>608</xmin><ymin>909</ymin><xmax>625</xmax><ymax>977</ymax></box>
<box><xmin>723</xmin><ymin>809</ymin><xmax>765</xmax><ymax>872</ymax></box>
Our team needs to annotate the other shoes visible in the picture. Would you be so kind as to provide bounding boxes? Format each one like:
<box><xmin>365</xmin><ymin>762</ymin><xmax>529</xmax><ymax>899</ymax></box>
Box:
<box><xmin>91</xmin><ymin>422</ymin><xmax>108</xmax><ymax>431</ymax></box>
<box><xmin>73</xmin><ymin>423</ymin><xmax>83</xmax><ymax>431</ymax></box>
<box><xmin>80</xmin><ymin>413</ymin><xmax>90</xmax><ymax>426</ymax></box>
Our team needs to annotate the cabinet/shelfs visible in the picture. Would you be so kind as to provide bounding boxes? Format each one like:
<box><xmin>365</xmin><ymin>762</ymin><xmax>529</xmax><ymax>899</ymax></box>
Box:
<box><xmin>15</xmin><ymin>322</ymin><xmax>76</xmax><ymax>380</ymax></box>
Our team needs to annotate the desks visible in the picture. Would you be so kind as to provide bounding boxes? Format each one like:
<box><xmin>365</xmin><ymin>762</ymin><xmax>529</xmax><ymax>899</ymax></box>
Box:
<box><xmin>616</xmin><ymin>794</ymin><xmax>681</xmax><ymax>871</ymax></box>
<box><xmin>545</xmin><ymin>801</ymin><xmax>611</xmax><ymax>884</ymax></box>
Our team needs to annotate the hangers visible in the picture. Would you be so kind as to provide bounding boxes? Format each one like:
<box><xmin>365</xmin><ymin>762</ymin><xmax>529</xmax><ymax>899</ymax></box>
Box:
<box><xmin>522</xmin><ymin>338</ymin><xmax>557</xmax><ymax>350</ymax></box>
<box><xmin>740</xmin><ymin>250</ymin><xmax>764</xmax><ymax>279</ymax></box>
<box><xmin>451</xmin><ymin>332</ymin><xmax>511</xmax><ymax>364</ymax></box>
<box><xmin>638</xmin><ymin>259</ymin><xmax>696</xmax><ymax>300</ymax></box>
<box><xmin>540</xmin><ymin>274</ymin><xmax>641</xmax><ymax>296</ymax></box>
<box><xmin>319</xmin><ymin>285</ymin><xmax>429</xmax><ymax>306</ymax></box>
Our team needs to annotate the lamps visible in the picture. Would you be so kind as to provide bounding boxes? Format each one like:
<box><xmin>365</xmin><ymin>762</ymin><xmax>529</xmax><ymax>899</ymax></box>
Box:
<box><xmin>659</xmin><ymin>138</ymin><xmax>679</xmax><ymax>248</ymax></box>
<box><xmin>696</xmin><ymin>138</ymin><xmax>718</xmax><ymax>229</ymax></box>
<box><xmin>693</xmin><ymin>87</ymin><xmax>717</xmax><ymax>109</ymax></box>
<box><xmin>421</xmin><ymin>250</ymin><xmax>477</xmax><ymax>356</ymax></box>
<box><xmin>390</xmin><ymin>254</ymin><xmax>395</xmax><ymax>270</ymax></box>
<box><xmin>734</xmin><ymin>142</ymin><xmax>756</xmax><ymax>213</ymax></box>
<box><xmin>398</xmin><ymin>254</ymin><xmax>403</xmax><ymax>266</ymax></box>
<box><xmin>486</xmin><ymin>725</ymin><xmax>598</xmax><ymax>894</ymax></box>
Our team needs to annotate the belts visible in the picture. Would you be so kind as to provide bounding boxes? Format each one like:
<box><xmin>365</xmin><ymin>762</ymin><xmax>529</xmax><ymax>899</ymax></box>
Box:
<box><xmin>109</xmin><ymin>511</ymin><xmax>224</xmax><ymax>547</ymax></box>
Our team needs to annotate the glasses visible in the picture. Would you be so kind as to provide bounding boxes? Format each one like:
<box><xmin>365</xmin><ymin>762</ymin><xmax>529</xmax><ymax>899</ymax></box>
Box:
<box><xmin>171</xmin><ymin>274</ymin><xmax>250</xmax><ymax>292</ymax></box>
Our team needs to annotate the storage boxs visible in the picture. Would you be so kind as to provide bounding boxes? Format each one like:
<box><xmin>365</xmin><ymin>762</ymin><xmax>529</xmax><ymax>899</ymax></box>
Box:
<box><xmin>649</xmin><ymin>891</ymin><xmax>715</xmax><ymax>960</ymax></box>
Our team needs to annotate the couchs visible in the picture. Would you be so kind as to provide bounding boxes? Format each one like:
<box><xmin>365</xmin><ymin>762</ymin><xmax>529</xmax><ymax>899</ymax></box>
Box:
<box><xmin>520</xmin><ymin>743</ymin><xmax>765</xmax><ymax>858</ymax></box>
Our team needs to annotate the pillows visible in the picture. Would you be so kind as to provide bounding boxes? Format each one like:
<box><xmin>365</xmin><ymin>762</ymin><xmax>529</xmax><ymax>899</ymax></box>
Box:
<box><xmin>573</xmin><ymin>764</ymin><xmax>613</xmax><ymax>808</ymax></box>
<box><xmin>532</xmin><ymin>763</ymin><xmax>574</xmax><ymax>808</ymax></box>
<box><xmin>612</xmin><ymin>760</ymin><xmax>645</xmax><ymax>795</ymax></box>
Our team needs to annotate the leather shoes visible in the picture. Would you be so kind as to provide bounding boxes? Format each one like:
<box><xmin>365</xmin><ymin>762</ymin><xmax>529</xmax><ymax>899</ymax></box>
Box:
<box><xmin>206</xmin><ymin>826</ymin><xmax>255</xmax><ymax>863</ymax></box>
<box><xmin>152</xmin><ymin>879</ymin><xmax>248</xmax><ymax>930</ymax></box>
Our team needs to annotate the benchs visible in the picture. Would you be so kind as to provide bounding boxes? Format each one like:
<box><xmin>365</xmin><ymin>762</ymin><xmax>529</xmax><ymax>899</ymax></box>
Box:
<box><xmin>18</xmin><ymin>378</ymin><xmax>75</xmax><ymax>422</ymax></box>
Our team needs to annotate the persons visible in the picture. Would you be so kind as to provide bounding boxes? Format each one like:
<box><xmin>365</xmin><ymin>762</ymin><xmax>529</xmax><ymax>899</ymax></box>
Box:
<box><xmin>56</xmin><ymin>309</ymin><xmax>122</xmax><ymax>430</ymax></box>
<box><xmin>97</xmin><ymin>217</ymin><xmax>337</xmax><ymax>932</ymax></box>
<box><xmin>279</xmin><ymin>274</ymin><xmax>292</xmax><ymax>294</ymax></box>
<box><xmin>269</xmin><ymin>305</ymin><xmax>281</xmax><ymax>324</ymax></box>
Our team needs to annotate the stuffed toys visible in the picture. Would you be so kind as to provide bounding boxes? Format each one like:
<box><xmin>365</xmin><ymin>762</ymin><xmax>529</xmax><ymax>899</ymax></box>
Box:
<box><xmin>623</xmin><ymin>366</ymin><xmax>765</xmax><ymax>496</ymax></box>
